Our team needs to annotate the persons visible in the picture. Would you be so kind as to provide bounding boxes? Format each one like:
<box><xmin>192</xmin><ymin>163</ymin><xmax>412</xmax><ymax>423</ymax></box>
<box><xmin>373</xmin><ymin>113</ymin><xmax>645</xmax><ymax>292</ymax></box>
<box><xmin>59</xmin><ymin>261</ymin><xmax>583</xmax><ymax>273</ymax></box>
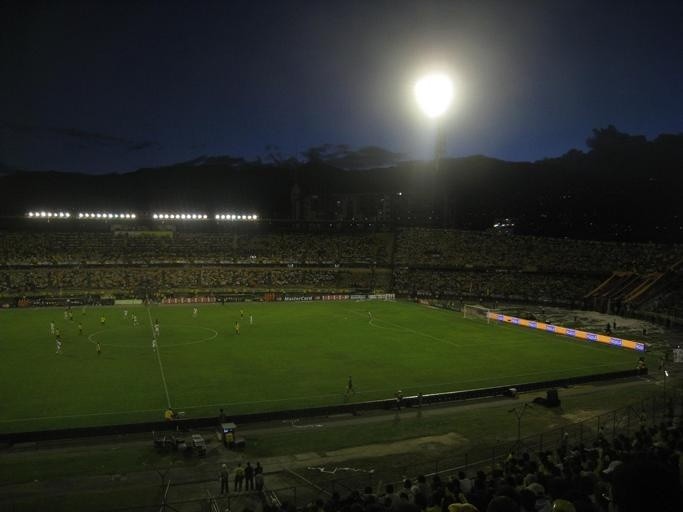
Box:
<box><xmin>164</xmin><ymin>408</ymin><xmax>174</xmax><ymax>422</ymax></box>
<box><xmin>641</xmin><ymin>327</ymin><xmax>646</xmax><ymax>336</ymax></box>
<box><xmin>345</xmin><ymin>375</ymin><xmax>355</xmax><ymax>398</ymax></box>
<box><xmin>604</xmin><ymin>322</ymin><xmax>610</xmax><ymax>334</ymax></box>
<box><xmin>55</xmin><ymin>327</ymin><xmax>60</xmax><ymax>337</ymax></box>
<box><xmin>151</xmin><ymin>337</ymin><xmax>157</xmax><ymax>353</ymax></box>
<box><xmin>612</xmin><ymin>320</ymin><xmax>616</xmax><ymax>330</ymax></box>
<box><xmin>49</xmin><ymin>320</ymin><xmax>55</xmax><ymax>335</ymax></box>
<box><xmin>78</xmin><ymin>323</ymin><xmax>83</xmax><ymax>335</ymax></box>
<box><xmin>153</xmin><ymin>321</ymin><xmax>160</xmax><ymax>337</ymax></box>
<box><xmin>0</xmin><ymin>226</ymin><xmax>681</xmax><ymax>325</ymax></box>
<box><xmin>214</xmin><ymin>409</ymin><xmax>681</xmax><ymax>511</ymax></box>
<box><xmin>414</xmin><ymin>391</ymin><xmax>424</xmax><ymax>410</ymax></box>
<box><xmin>392</xmin><ymin>389</ymin><xmax>403</xmax><ymax>410</ymax></box>
<box><xmin>94</xmin><ymin>342</ymin><xmax>102</xmax><ymax>356</ymax></box>
<box><xmin>232</xmin><ymin>321</ymin><xmax>239</xmax><ymax>336</ymax></box>
<box><xmin>55</xmin><ymin>338</ymin><xmax>62</xmax><ymax>355</ymax></box>
